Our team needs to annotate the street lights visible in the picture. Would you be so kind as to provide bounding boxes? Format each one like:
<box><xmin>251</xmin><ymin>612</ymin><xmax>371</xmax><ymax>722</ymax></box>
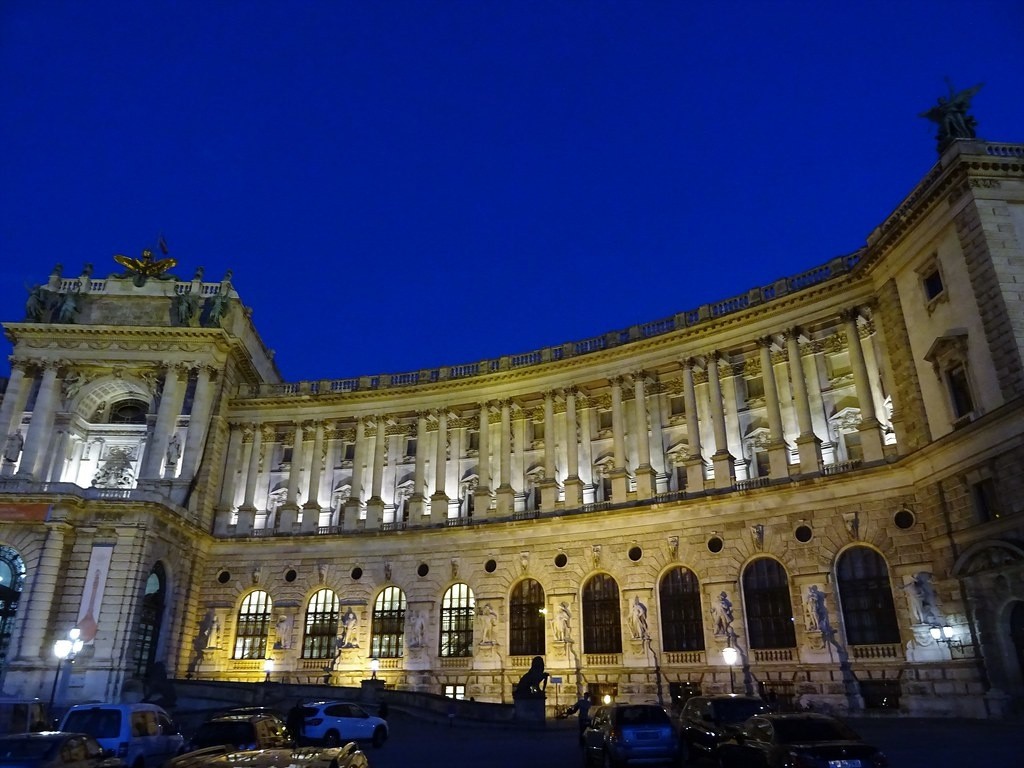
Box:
<box><xmin>44</xmin><ymin>631</ymin><xmax>73</xmax><ymax>719</ymax></box>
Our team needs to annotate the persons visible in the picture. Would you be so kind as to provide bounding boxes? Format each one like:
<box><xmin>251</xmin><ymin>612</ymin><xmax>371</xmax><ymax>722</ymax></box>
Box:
<box><xmin>895</xmin><ymin>572</ymin><xmax>944</xmax><ymax>624</ymax></box>
<box><xmin>210</xmin><ymin>286</ymin><xmax>231</xmax><ymax>329</ymax></box>
<box><xmin>478</xmin><ymin>604</ymin><xmax>497</xmax><ymax>645</ymax></box>
<box><xmin>168</xmin><ymin>432</ymin><xmax>181</xmax><ymax>464</ymax></box>
<box><xmin>173</xmin><ymin>285</ymin><xmax>198</xmax><ymax>327</ymax></box>
<box><xmin>551</xmin><ymin>604</ymin><xmax>570</xmax><ymax>642</ymax></box>
<box><xmin>24</xmin><ymin>283</ymin><xmax>79</xmax><ymax>323</ymax></box>
<box><xmin>5</xmin><ymin>428</ymin><xmax>23</xmax><ymax>462</ymax></box>
<box><xmin>569</xmin><ymin>692</ymin><xmax>595</xmax><ymax>733</ymax></box>
<box><xmin>341</xmin><ymin>613</ymin><xmax>357</xmax><ymax>649</ymax></box>
<box><xmin>709</xmin><ymin>591</ymin><xmax>732</xmax><ymax>636</ymax></box>
<box><xmin>204</xmin><ymin>616</ymin><xmax>221</xmax><ymax>650</ymax></box>
<box><xmin>285</xmin><ymin>696</ymin><xmax>305</xmax><ymax>748</ymax></box>
<box><xmin>803</xmin><ymin>586</ymin><xmax>822</xmax><ymax>632</ymax></box>
<box><xmin>626</xmin><ymin>598</ymin><xmax>645</xmax><ymax>639</ymax></box>
<box><xmin>275</xmin><ymin>614</ymin><xmax>296</xmax><ymax>650</ymax></box>
<box><xmin>409</xmin><ymin>611</ymin><xmax>426</xmax><ymax>647</ymax></box>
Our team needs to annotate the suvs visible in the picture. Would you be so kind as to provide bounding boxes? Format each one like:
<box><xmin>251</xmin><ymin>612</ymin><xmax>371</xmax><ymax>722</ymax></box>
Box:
<box><xmin>158</xmin><ymin>741</ymin><xmax>370</xmax><ymax>768</ymax></box>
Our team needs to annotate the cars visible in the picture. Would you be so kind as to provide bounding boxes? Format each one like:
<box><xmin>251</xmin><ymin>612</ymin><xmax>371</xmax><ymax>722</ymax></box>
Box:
<box><xmin>727</xmin><ymin>711</ymin><xmax>890</xmax><ymax>768</ymax></box>
<box><xmin>581</xmin><ymin>699</ymin><xmax>683</xmax><ymax>768</ymax></box>
<box><xmin>0</xmin><ymin>730</ymin><xmax>125</xmax><ymax>768</ymax></box>
<box><xmin>59</xmin><ymin>701</ymin><xmax>187</xmax><ymax>768</ymax></box>
<box><xmin>676</xmin><ymin>693</ymin><xmax>779</xmax><ymax>768</ymax></box>
<box><xmin>288</xmin><ymin>697</ymin><xmax>391</xmax><ymax>750</ymax></box>
<box><xmin>194</xmin><ymin>704</ymin><xmax>299</xmax><ymax>752</ymax></box>
